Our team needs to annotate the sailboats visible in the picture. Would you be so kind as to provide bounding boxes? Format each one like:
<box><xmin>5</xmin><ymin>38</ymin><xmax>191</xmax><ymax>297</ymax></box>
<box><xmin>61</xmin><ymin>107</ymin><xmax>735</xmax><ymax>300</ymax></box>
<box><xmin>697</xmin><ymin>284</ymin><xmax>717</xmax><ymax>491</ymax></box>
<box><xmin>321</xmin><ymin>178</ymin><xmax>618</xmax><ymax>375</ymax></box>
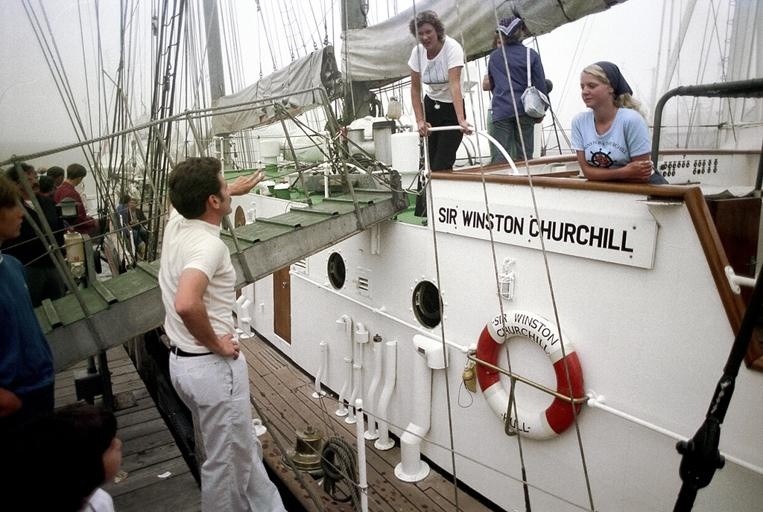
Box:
<box><xmin>17</xmin><ymin>0</ymin><xmax>763</xmax><ymax>512</ymax></box>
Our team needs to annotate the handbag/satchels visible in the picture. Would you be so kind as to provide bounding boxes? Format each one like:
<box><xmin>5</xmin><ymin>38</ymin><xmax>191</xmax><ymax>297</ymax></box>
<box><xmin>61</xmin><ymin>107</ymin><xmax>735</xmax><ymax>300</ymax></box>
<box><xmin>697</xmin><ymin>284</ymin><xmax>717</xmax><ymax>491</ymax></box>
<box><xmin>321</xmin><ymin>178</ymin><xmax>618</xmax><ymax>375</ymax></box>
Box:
<box><xmin>520</xmin><ymin>85</ymin><xmax>550</xmax><ymax>119</ymax></box>
<box><xmin>171</xmin><ymin>345</ymin><xmax>214</xmax><ymax>357</ymax></box>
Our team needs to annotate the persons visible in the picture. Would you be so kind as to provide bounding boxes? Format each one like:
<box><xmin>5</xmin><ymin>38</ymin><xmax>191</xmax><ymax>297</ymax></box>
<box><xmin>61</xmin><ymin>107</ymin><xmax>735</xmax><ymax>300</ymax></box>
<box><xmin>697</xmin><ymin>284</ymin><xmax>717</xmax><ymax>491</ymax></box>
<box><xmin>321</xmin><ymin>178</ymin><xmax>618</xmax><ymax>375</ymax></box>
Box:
<box><xmin>118</xmin><ymin>196</ymin><xmax>132</xmax><ymax>241</ymax></box>
<box><xmin>1</xmin><ymin>177</ymin><xmax>56</xmax><ymax>411</ymax></box>
<box><xmin>487</xmin><ymin>14</ymin><xmax>553</xmax><ymax>164</ymax></box>
<box><xmin>0</xmin><ymin>162</ymin><xmax>103</xmax><ymax>301</ymax></box>
<box><xmin>157</xmin><ymin>153</ymin><xmax>288</xmax><ymax>512</ymax></box>
<box><xmin>408</xmin><ymin>9</ymin><xmax>475</xmax><ymax>172</ymax></box>
<box><xmin>23</xmin><ymin>403</ymin><xmax>123</xmax><ymax>512</ymax></box>
<box><xmin>569</xmin><ymin>63</ymin><xmax>668</xmax><ymax>187</ymax></box>
<box><xmin>128</xmin><ymin>199</ymin><xmax>150</xmax><ymax>244</ymax></box>
<box><xmin>483</xmin><ymin>34</ymin><xmax>519</xmax><ymax>163</ymax></box>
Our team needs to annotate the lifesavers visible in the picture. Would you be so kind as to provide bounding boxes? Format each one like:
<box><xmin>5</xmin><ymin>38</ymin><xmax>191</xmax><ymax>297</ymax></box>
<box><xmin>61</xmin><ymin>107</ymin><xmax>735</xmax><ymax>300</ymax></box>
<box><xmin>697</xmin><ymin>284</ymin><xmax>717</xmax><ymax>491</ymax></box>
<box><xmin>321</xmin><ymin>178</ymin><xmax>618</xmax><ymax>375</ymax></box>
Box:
<box><xmin>476</xmin><ymin>313</ymin><xmax>584</xmax><ymax>440</ymax></box>
